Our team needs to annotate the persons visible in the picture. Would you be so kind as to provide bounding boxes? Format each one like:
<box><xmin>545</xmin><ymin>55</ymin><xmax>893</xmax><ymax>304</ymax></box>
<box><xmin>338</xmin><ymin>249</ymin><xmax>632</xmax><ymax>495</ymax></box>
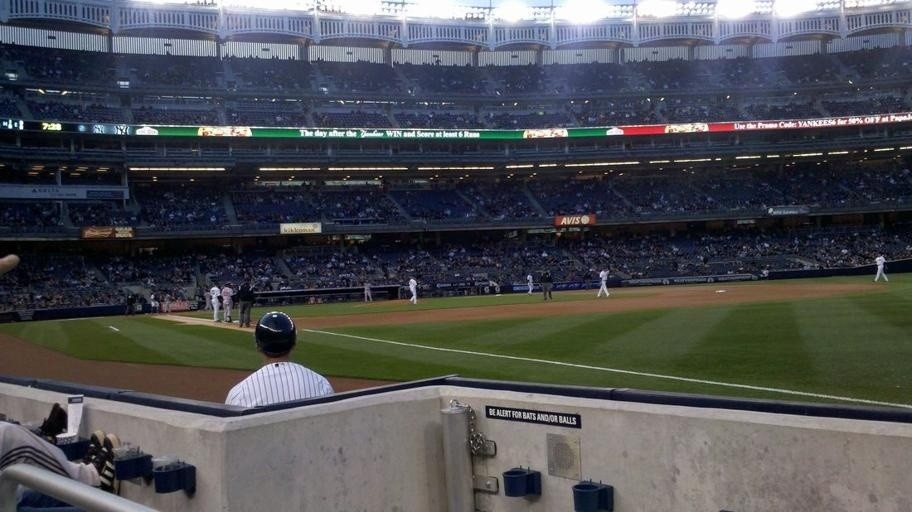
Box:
<box><xmin>0</xmin><ymin>420</ymin><xmax>123</xmax><ymax>512</ymax></box>
<box><xmin>225</xmin><ymin>311</ymin><xmax>335</xmax><ymax>409</ymax></box>
<box><xmin>0</xmin><ymin>402</ymin><xmax>68</xmax><ymax>444</ymax></box>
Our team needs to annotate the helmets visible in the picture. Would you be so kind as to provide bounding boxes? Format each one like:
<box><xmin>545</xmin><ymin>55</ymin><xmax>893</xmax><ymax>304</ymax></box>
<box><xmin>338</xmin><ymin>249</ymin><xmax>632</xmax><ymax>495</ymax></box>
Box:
<box><xmin>255</xmin><ymin>312</ymin><xmax>298</xmax><ymax>355</ymax></box>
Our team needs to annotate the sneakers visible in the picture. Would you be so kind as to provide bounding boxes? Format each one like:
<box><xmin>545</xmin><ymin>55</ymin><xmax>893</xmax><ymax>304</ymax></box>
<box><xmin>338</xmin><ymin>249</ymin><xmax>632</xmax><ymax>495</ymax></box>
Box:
<box><xmin>40</xmin><ymin>401</ymin><xmax>67</xmax><ymax>446</ymax></box>
<box><xmin>84</xmin><ymin>430</ymin><xmax>120</xmax><ymax>496</ymax></box>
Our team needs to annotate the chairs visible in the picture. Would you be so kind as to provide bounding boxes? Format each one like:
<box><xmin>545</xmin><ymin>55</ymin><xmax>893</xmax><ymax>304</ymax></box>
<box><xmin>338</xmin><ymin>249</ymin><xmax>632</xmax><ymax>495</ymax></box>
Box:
<box><xmin>1</xmin><ymin>47</ymin><xmax>467</xmax><ymax>315</ymax></box>
<box><xmin>468</xmin><ymin>52</ymin><xmax>912</xmax><ymax>286</ymax></box>
